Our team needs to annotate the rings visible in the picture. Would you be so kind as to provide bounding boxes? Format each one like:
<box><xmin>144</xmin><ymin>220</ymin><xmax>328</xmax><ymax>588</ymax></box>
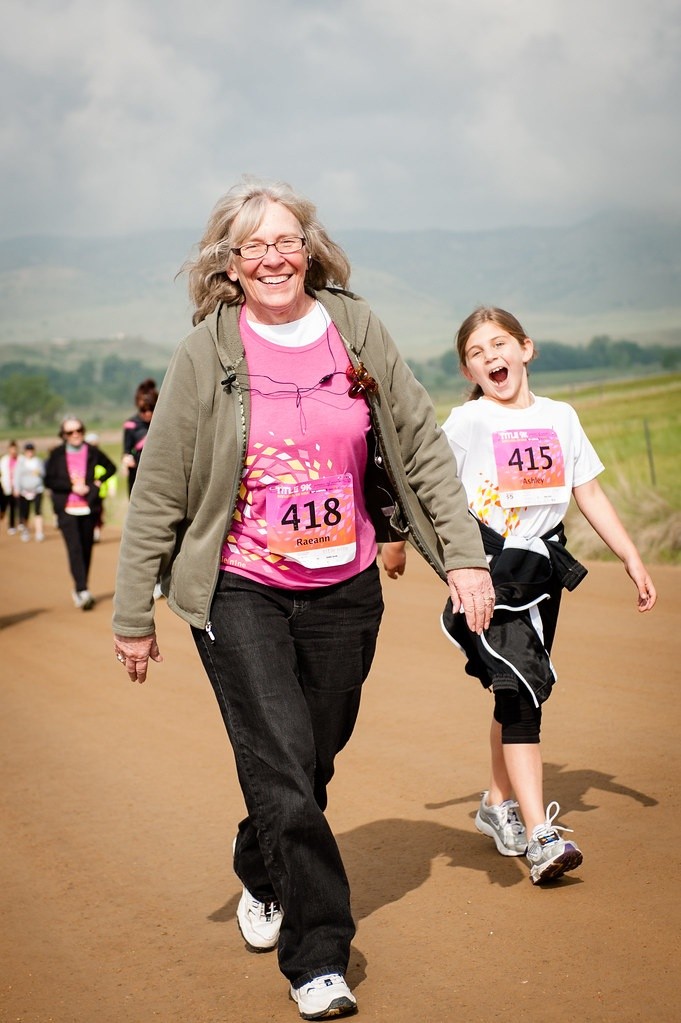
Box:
<box><xmin>486</xmin><ymin>596</ymin><xmax>493</xmax><ymax>600</ymax></box>
<box><xmin>117</xmin><ymin>655</ymin><xmax>124</xmax><ymax>663</ymax></box>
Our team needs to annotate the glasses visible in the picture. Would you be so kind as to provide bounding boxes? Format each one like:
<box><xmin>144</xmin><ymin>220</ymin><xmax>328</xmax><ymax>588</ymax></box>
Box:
<box><xmin>140</xmin><ymin>406</ymin><xmax>154</xmax><ymax>413</ymax></box>
<box><xmin>231</xmin><ymin>236</ymin><xmax>305</xmax><ymax>259</ymax></box>
<box><xmin>65</xmin><ymin>428</ymin><xmax>83</xmax><ymax>436</ymax></box>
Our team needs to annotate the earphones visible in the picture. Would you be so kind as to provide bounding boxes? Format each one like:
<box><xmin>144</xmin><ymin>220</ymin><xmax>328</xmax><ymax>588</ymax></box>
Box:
<box><xmin>308</xmin><ymin>252</ymin><xmax>313</xmax><ymax>264</ymax></box>
<box><xmin>374</xmin><ymin>456</ymin><xmax>383</xmax><ymax>464</ymax></box>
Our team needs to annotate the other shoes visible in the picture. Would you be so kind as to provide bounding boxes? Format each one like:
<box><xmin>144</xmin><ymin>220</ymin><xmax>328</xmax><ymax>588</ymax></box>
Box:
<box><xmin>76</xmin><ymin>590</ymin><xmax>94</xmax><ymax>609</ymax></box>
<box><xmin>153</xmin><ymin>583</ymin><xmax>163</xmax><ymax>599</ymax></box>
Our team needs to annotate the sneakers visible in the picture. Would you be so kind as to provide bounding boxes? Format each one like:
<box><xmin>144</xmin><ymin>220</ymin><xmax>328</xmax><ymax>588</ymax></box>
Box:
<box><xmin>290</xmin><ymin>972</ymin><xmax>358</xmax><ymax>1018</ymax></box>
<box><xmin>526</xmin><ymin>800</ymin><xmax>583</xmax><ymax>885</ymax></box>
<box><xmin>233</xmin><ymin>837</ymin><xmax>284</xmax><ymax>949</ymax></box>
<box><xmin>474</xmin><ymin>791</ymin><xmax>526</xmax><ymax>857</ymax></box>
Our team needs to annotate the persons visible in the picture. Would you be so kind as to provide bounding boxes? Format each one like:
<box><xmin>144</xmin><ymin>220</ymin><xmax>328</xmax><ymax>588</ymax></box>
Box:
<box><xmin>380</xmin><ymin>307</ymin><xmax>656</xmax><ymax>884</ymax></box>
<box><xmin>112</xmin><ymin>180</ymin><xmax>496</xmax><ymax>1021</ymax></box>
<box><xmin>0</xmin><ymin>440</ymin><xmax>60</xmax><ymax>543</ymax></box>
<box><xmin>43</xmin><ymin>417</ymin><xmax>117</xmax><ymax>610</ymax></box>
<box><xmin>124</xmin><ymin>379</ymin><xmax>191</xmax><ymax>602</ymax></box>
<box><xmin>84</xmin><ymin>432</ymin><xmax>117</xmax><ymax>544</ymax></box>
